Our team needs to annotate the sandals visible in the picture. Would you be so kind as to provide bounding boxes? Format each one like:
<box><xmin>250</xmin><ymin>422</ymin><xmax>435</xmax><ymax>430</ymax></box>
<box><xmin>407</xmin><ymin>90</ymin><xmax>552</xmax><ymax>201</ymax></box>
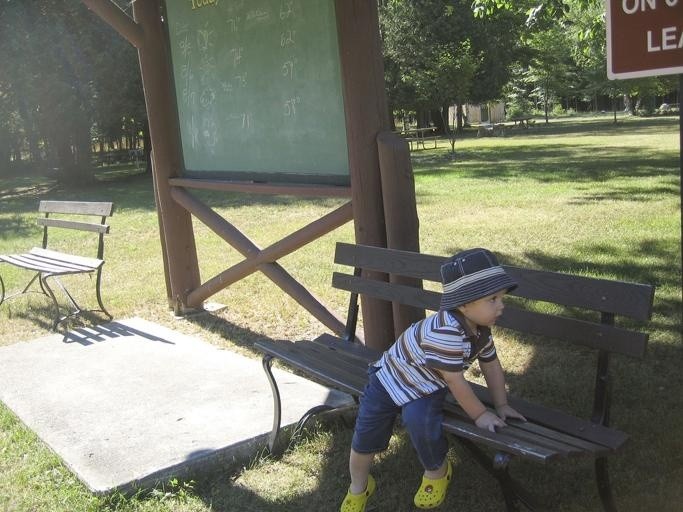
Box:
<box><xmin>413</xmin><ymin>457</ymin><xmax>453</xmax><ymax>510</ymax></box>
<box><xmin>340</xmin><ymin>474</ymin><xmax>376</xmax><ymax>512</ymax></box>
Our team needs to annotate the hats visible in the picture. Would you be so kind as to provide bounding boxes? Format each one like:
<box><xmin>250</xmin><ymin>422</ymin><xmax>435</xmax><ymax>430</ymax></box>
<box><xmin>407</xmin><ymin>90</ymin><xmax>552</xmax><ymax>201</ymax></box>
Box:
<box><xmin>438</xmin><ymin>246</ymin><xmax>518</xmax><ymax>314</ymax></box>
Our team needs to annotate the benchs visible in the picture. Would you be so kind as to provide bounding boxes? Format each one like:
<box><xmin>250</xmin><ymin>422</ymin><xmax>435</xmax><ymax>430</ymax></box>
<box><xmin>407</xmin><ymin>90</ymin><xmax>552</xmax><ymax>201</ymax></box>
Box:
<box><xmin>253</xmin><ymin>242</ymin><xmax>657</xmax><ymax>512</ymax></box>
<box><xmin>0</xmin><ymin>200</ymin><xmax>116</xmax><ymax>333</ymax></box>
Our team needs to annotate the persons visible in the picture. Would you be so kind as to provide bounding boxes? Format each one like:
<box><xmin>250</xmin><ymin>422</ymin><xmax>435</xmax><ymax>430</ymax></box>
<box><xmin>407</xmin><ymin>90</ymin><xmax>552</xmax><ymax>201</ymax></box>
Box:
<box><xmin>341</xmin><ymin>247</ymin><xmax>528</xmax><ymax>512</ymax></box>
